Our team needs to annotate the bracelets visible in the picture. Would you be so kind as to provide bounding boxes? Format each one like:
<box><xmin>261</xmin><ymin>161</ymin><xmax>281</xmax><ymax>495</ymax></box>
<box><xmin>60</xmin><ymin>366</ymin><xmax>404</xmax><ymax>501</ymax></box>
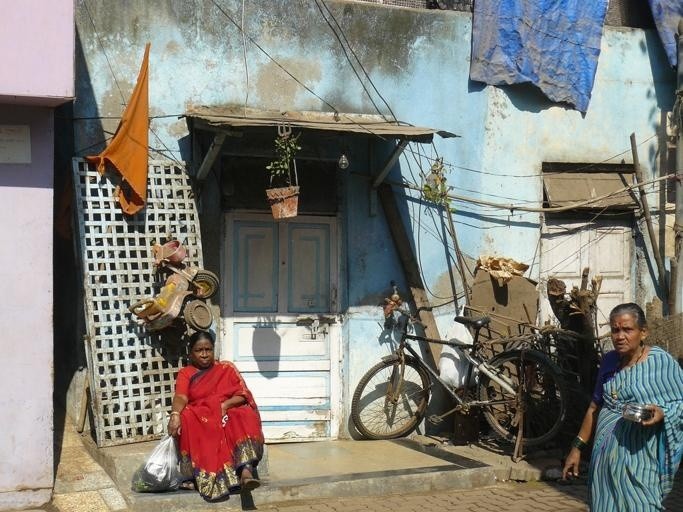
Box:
<box><xmin>170</xmin><ymin>411</ymin><xmax>180</xmax><ymax>415</ymax></box>
<box><xmin>571</xmin><ymin>435</ymin><xmax>587</xmax><ymax>452</ymax></box>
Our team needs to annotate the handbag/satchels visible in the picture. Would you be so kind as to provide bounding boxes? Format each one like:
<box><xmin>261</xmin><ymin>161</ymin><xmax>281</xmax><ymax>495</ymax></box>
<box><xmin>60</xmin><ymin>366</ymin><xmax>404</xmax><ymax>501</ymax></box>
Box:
<box><xmin>132</xmin><ymin>434</ymin><xmax>181</xmax><ymax>493</ymax></box>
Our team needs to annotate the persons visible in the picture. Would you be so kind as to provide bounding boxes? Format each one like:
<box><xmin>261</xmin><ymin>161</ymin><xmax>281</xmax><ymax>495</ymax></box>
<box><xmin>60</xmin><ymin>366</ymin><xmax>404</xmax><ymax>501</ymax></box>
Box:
<box><xmin>167</xmin><ymin>331</ymin><xmax>265</xmax><ymax>502</ymax></box>
<box><xmin>562</xmin><ymin>303</ymin><xmax>683</xmax><ymax>512</ymax></box>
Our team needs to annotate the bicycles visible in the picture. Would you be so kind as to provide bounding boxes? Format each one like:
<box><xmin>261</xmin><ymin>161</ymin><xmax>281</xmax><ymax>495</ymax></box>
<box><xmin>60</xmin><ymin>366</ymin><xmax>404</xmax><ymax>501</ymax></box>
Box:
<box><xmin>349</xmin><ymin>294</ymin><xmax>573</xmax><ymax>463</ymax></box>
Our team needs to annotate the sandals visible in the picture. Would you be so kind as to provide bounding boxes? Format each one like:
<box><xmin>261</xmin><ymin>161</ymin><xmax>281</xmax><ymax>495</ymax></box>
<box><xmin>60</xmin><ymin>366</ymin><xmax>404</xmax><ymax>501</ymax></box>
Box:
<box><xmin>240</xmin><ymin>476</ymin><xmax>260</xmax><ymax>493</ymax></box>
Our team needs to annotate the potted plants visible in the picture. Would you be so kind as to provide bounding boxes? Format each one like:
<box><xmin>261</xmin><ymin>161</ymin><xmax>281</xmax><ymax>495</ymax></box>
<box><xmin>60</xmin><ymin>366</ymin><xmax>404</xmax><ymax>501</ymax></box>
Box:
<box><xmin>265</xmin><ymin>131</ymin><xmax>302</xmax><ymax>221</ymax></box>
